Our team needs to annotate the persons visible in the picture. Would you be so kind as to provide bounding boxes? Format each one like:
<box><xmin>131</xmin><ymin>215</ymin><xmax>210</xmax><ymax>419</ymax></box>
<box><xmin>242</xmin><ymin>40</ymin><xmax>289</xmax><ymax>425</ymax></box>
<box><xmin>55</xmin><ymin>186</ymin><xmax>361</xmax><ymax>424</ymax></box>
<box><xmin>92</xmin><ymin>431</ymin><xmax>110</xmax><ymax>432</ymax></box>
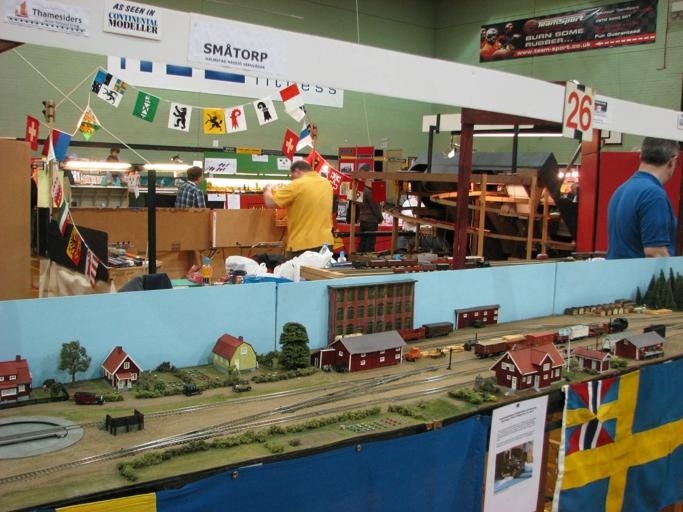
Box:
<box><xmin>262</xmin><ymin>161</ymin><xmax>331</xmax><ymax>258</ymax></box>
<box><xmin>394</xmin><ymin>192</ymin><xmax>426</xmax><ymax>250</ymax></box>
<box><xmin>175</xmin><ymin>167</ymin><xmax>204</xmax><ymax>207</ymax></box>
<box><xmin>354</xmin><ymin>165</ymin><xmax>383</xmax><ymax>253</ymax></box>
<box><xmin>480</xmin><ymin>22</ymin><xmax>523</xmax><ymax>62</ymax></box>
<box><xmin>602</xmin><ymin>134</ymin><xmax>678</xmax><ymax>258</ymax></box>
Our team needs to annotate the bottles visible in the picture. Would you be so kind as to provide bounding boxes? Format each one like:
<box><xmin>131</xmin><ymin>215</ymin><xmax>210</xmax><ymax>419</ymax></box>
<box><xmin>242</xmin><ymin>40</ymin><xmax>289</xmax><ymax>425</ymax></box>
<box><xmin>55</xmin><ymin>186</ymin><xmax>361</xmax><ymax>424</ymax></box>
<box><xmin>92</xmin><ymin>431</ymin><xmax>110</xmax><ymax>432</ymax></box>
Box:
<box><xmin>319</xmin><ymin>242</ymin><xmax>346</xmax><ymax>269</ymax></box>
<box><xmin>200</xmin><ymin>256</ymin><xmax>214</xmax><ymax>287</ymax></box>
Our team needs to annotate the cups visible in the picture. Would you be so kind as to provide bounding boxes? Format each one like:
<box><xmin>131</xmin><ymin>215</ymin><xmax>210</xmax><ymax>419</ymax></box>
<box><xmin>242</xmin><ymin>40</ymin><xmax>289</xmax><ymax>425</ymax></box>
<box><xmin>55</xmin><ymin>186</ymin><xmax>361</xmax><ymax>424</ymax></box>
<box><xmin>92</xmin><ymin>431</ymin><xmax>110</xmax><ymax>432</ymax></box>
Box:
<box><xmin>232</xmin><ymin>269</ymin><xmax>247</xmax><ymax>284</ymax></box>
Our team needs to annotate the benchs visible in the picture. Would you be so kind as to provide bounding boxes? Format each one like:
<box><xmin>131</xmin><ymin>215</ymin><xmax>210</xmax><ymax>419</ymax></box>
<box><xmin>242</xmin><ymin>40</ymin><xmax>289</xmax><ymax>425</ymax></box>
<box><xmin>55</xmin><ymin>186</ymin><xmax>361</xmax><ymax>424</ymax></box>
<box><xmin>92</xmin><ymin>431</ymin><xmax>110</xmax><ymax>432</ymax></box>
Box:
<box><xmin>106</xmin><ymin>409</ymin><xmax>144</xmax><ymax>436</ymax></box>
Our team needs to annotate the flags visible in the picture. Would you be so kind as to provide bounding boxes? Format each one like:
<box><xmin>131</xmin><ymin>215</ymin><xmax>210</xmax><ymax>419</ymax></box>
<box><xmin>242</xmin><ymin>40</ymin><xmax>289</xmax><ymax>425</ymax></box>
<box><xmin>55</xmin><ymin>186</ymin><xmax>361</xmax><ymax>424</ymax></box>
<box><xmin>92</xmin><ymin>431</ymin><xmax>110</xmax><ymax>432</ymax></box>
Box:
<box><xmin>282</xmin><ymin>127</ymin><xmax>385</xmax><ymax>203</ymax></box>
<box><xmin>88</xmin><ymin>66</ymin><xmax>307</xmax><ymax>156</ymax></box>
<box><xmin>21</xmin><ymin>105</ymin><xmax>98</xmax><ymax>287</ymax></box>
